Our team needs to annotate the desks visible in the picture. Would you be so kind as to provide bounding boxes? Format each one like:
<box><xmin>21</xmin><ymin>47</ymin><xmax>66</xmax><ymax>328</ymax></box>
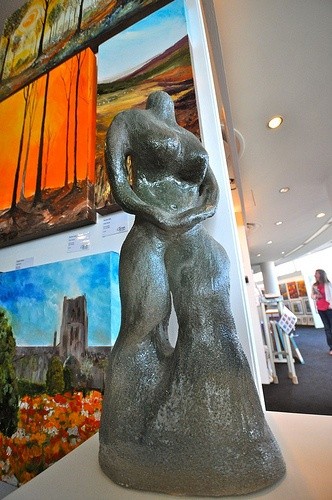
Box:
<box><xmin>0</xmin><ymin>411</ymin><xmax>332</xmax><ymax>500</ymax></box>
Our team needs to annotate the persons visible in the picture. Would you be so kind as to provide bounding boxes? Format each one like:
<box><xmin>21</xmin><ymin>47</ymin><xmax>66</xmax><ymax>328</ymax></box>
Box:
<box><xmin>311</xmin><ymin>269</ymin><xmax>332</xmax><ymax>355</ymax></box>
<box><xmin>99</xmin><ymin>89</ymin><xmax>285</xmax><ymax>497</ymax></box>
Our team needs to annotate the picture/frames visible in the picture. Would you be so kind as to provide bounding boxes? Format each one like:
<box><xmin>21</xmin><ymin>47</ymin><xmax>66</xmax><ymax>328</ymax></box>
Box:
<box><xmin>284</xmin><ymin>298</ymin><xmax>312</xmax><ymax>315</ymax></box>
